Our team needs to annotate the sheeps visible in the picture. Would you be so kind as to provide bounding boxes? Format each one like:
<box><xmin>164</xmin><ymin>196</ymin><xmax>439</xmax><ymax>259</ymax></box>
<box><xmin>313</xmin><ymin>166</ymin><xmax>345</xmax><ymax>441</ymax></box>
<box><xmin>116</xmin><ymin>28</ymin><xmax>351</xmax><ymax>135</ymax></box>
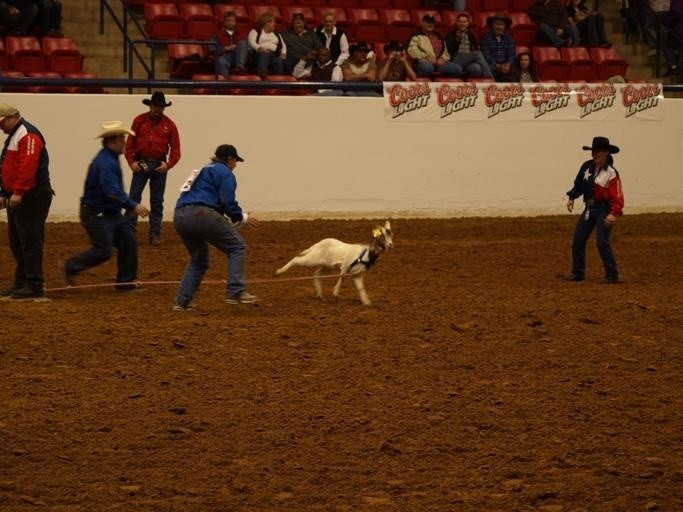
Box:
<box><xmin>273</xmin><ymin>220</ymin><xmax>394</xmax><ymax>308</ymax></box>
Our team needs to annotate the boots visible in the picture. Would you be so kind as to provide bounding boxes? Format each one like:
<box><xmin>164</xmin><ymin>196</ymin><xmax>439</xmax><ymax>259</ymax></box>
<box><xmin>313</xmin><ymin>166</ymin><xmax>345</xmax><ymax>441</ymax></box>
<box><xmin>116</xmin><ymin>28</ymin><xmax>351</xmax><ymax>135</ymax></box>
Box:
<box><xmin>1</xmin><ymin>281</ymin><xmax>45</xmax><ymax>298</ymax></box>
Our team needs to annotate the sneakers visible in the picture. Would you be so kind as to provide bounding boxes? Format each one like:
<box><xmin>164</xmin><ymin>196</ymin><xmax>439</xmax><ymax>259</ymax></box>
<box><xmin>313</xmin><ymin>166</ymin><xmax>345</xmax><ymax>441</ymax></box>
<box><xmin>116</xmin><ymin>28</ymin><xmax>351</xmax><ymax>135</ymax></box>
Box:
<box><xmin>172</xmin><ymin>298</ymin><xmax>201</xmax><ymax>313</ymax></box>
<box><xmin>224</xmin><ymin>291</ymin><xmax>258</xmax><ymax>305</ymax></box>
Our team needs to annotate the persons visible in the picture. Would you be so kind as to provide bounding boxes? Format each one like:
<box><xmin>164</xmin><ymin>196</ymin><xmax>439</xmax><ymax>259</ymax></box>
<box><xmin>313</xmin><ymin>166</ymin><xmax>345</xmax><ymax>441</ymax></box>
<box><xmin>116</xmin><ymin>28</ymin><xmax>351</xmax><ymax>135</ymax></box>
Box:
<box><xmin>528</xmin><ymin>0</ymin><xmax>574</xmax><ymax>49</ymax></box>
<box><xmin>567</xmin><ymin>0</ymin><xmax>614</xmax><ymax>49</ymax></box>
<box><xmin>517</xmin><ymin>50</ymin><xmax>540</xmax><ymax>84</ymax></box>
<box><xmin>563</xmin><ymin>136</ymin><xmax>625</xmax><ymax>287</ymax></box>
<box><xmin>478</xmin><ymin>10</ymin><xmax>522</xmax><ymax>82</ymax></box>
<box><xmin>619</xmin><ymin>1</ymin><xmax>683</xmax><ymax>77</ymax></box>
<box><xmin>0</xmin><ymin>103</ymin><xmax>56</xmax><ymax>299</ymax></box>
<box><xmin>407</xmin><ymin>13</ymin><xmax>465</xmax><ymax>80</ymax></box>
<box><xmin>444</xmin><ymin>12</ymin><xmax>498</xmax><ymax>82</ymax></box>
<box><xmin>280</xmin><ymin>10</ymin><xmax>325</xmax><ymax>74</ymax></box>
<box><xmin>124</xmin><ymin>90</ymin><xmax>181</xmax><ymax>247</ymax></box>
<box><xmin>312</xmin><ymin>12</ymin><xmax>352</xmax><ymax>67</ymax></box>
<box><xmin>292</xmin><ymin>38</ymin><xmax>417</xmax><ymax>82</ymax></box>
<box><xmin>246</xmin><ymin>10</ymin><xmax>287</xmax><ymax>83</ymax></box>
<box><xmin>209</xmin><ymin>11</ymin><xmax>250</xmax><ymax>82</ymax></box>
<box><xmin>173</xmin><ymin>144</ymin><xmax>262</xmax><ymax>311</ymax></box>
<box><xmin>63</xmin><ymin>117</ymin><xmax>152</xmax><ymax>291</ymax></box>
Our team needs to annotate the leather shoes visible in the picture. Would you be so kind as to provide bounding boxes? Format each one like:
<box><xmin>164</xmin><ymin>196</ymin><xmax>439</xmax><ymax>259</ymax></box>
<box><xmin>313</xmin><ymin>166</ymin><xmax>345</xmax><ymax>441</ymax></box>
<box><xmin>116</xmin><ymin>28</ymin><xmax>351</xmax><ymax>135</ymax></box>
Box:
<box><xmin>566</xmin><ymin>272</ymin><xmax>585</xmax><ymax>282</ymax></box>
<box><xmin>600</xmin><ymin>275</ymin><xmax>619</xmax><ymax>284</ymax></box>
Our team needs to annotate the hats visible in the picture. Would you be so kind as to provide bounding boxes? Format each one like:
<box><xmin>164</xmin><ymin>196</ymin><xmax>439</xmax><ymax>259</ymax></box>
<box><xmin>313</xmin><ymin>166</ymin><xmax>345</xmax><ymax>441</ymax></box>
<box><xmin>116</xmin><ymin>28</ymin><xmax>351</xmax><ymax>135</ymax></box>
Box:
<box><xmin>216</xmin><ymin>144</ymin><xmax>247</xmax><ymax>161</ymax></box>
<box><xmin>1</xmin><ymin>101</ymin><xmax>20</xmax><ymax>120</ymax></box>
<box><xmin>348</xmin><ymin>39</ymin><xmax>375</xmax><ymax>53</ymax></box>
<box><xmin>420</xmin><ymin>12</ymin><xmax>436</xmax><ymax>26</ymax></box>
<box><xmin>142</xmin><ymin>90</ymin><xmax>172</xmax><ymax>107</ymax></box>
<box><xmin>384</xmin><ymin>40</ymin><xmax>405</xmax><ymax>55</ymax></box>
<box><xmin>583</xmin><ymin>136</ymin><xmax>620</xmax><ymax>156</ymax></box>
<box><xmin>487</xmin><ymin>12</ymin><xmax>512</xmax><ymax>24</ymax></box>
<box><xmin>93</xmin><ymin>120</ymin><xmax>137</xmax><ymax>140</ymax></box>
